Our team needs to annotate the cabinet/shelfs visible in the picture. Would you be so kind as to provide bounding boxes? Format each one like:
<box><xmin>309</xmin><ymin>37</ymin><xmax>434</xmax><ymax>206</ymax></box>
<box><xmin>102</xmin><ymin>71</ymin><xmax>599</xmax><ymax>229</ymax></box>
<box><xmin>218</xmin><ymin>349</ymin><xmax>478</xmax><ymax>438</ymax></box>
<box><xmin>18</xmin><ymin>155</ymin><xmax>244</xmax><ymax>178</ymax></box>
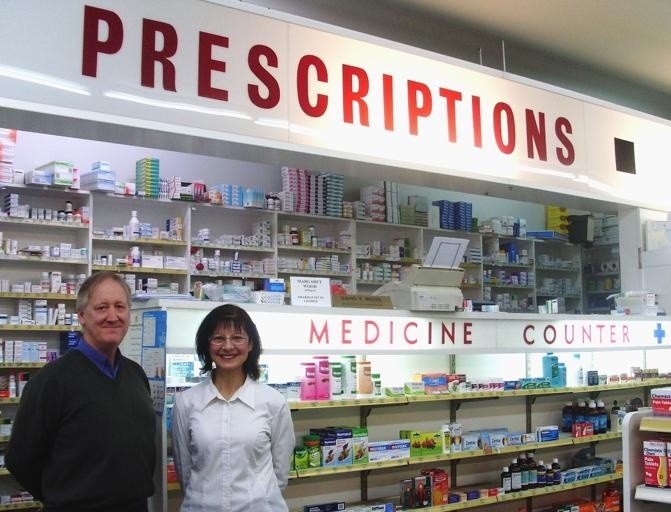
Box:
<box><xmin>277</xmin><ymin>210</ymin><xmax>355</xmax><ymax>307</ymax></box>
<box><xmin>2</xmin><ymin>182</ymin><xmax>92</xmax><ymax>512</ymax></box>
<box><xmin>582</xmin><ymin>243</ymin><xmax>619</xmax><ymax>315</ymax></box>
<box><xmin>483</xmin><ymin>235</ymin><xmax>535</xmax><ymax>314</ymax></box>
<box><xmin>91</xmin><ymin>190</ymin><xmax>191</xmax><ymax>305</ymax></box>
<box><xmin>535</xmin><ymin>239</ymin><xmax>583</xmax><ymax>316</ymax></box>
<box><xmin>358</xmin><ymin>220</ymin><xmax>424</xmax><ymax>296</ymax></box>
<box><xmin>423</xmin><ymin>226</ymin><xmax>483</xmax><ymax>311</ymax></box>
<box><xmin>119</xmin><ymin>306</ymin><xmax>670</xmax><ymax>512</ymax></box>
<box><xmin>189</xmin><ymin>209</ymin><xmax>278</xmax><ymax>302</ymax></box>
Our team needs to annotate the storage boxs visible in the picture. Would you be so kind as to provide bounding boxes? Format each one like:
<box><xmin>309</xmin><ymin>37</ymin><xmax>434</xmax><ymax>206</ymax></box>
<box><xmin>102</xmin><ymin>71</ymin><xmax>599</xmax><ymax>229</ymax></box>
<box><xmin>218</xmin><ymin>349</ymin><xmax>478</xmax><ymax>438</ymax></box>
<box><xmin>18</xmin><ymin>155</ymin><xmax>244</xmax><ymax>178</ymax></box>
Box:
<box><xmin>23</xmin><ymin>157</ymin><xmax>622</xmax><ymax>232</ymax></box>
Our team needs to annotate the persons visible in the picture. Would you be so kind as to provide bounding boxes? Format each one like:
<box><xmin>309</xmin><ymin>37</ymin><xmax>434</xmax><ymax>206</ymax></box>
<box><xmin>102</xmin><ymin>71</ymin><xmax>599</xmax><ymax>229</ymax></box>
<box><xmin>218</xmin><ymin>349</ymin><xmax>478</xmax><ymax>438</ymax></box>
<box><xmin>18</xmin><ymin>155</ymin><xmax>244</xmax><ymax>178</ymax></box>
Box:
<box><xmin>172</xmin><ymin>305</ymin><xmax>295</xmax><ymax>512</ymax></box>
<box><xmin>4</xmin><ymin>271</ymin><xmax>158</xmax><ymax>512</ymax></box>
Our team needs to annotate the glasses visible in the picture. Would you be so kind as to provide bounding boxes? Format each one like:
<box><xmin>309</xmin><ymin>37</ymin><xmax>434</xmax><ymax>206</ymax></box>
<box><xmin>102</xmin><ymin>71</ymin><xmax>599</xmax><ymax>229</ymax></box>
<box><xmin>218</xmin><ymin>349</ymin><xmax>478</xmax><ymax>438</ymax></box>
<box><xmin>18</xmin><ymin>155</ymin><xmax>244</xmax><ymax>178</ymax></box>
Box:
<box><xmin>209</xmin><ymin>334</ymin><xmax>249</xmax><ymax>345</ymax></box>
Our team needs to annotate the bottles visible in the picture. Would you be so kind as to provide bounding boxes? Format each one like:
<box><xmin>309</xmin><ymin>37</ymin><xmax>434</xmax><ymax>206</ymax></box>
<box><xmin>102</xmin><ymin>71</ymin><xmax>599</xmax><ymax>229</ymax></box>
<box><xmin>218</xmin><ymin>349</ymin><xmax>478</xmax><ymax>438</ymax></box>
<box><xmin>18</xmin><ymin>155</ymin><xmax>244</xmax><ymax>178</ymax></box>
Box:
<box><xmin>300</xmin><ymin>354</ymin><xmax>382</xmax><ymax>399</ymax></box>
<box><xmin>267</xmin><ymin>191</ymin><xmax>280</xmax><ymax>210</ymax></box>
<box><xmin>303</xmin><ymin>435</ymin><xmax>322</xmax><ymax>468</ymax></box>
<box><xmin>500</xmin><ymin>453</ymin><xmax>562</xmax><ymax>493</ymax></box>
<box><xmin>194</xmin><ymin>229</ymin><xmax>231</xmax><ymax>273</ymax></box>
<box><xmin>289</xmin><ymin>225</ymin><xmax>318</xmax><ymax>248</ymax></box>
<box><xmin>40</xmin><ymin>201</ymin><xmax>140</xmax><ymax>326</ymax></box>
<box><xmin>543</xmin><ymin>351</ymin><xmax>639</xmax><ymax>437</ymax></box>
